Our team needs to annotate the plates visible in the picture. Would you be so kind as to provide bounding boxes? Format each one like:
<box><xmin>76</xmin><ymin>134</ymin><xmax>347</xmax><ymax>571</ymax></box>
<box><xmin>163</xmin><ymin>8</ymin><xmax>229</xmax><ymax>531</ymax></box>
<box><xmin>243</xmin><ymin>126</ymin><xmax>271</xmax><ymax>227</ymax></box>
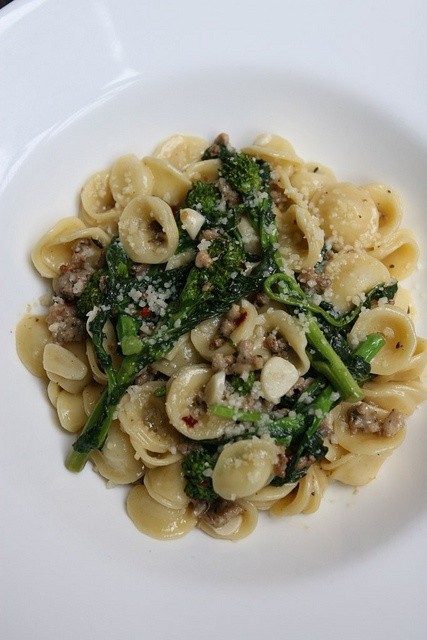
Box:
<box><xmin>0</xmin><ymin>0</ymin><xmax>427</xmax><ymax>640</ymax></box>
